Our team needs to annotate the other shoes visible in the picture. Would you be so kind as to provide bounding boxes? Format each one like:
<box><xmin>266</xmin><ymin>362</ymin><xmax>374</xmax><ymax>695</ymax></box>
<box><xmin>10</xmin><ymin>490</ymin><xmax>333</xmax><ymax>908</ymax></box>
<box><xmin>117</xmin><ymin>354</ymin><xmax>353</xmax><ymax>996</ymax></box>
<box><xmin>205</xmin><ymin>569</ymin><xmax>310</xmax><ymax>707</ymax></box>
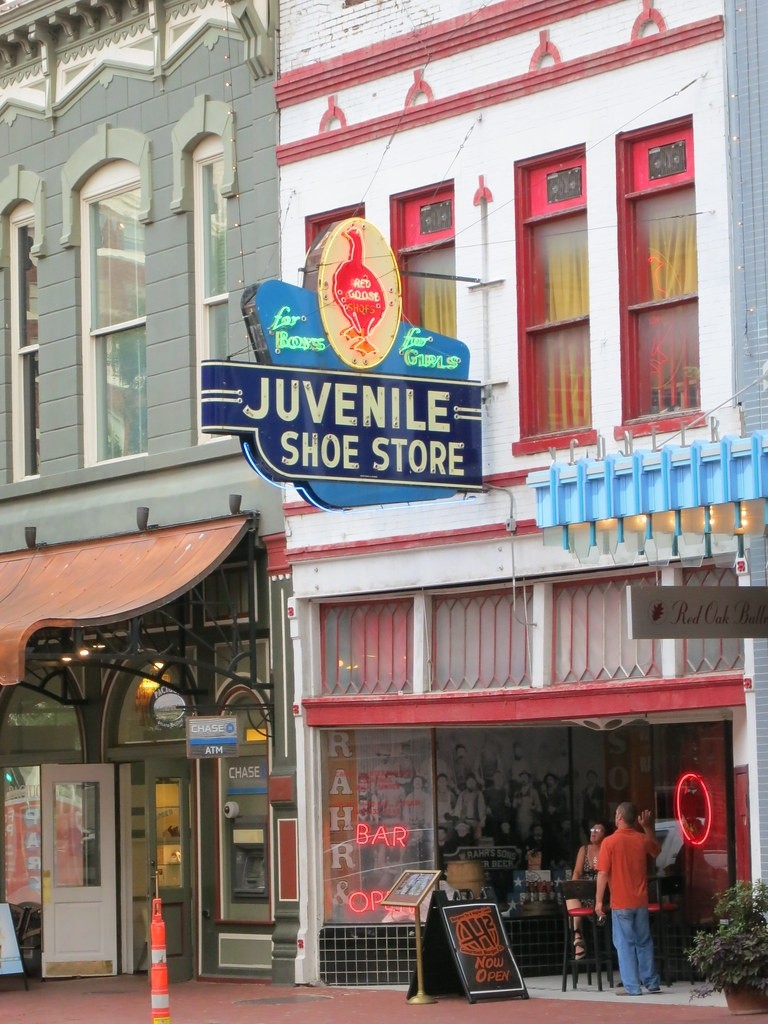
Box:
<box><xmin>649</xmin><ymin>986</ymin><xmax>662</xmax><ymax>993</ymax></box>
<box><xmin>574</xmin><ymin>929</ymin><xmax>587</xmax><ymax>961</ymax></box>
<box><xmin>616</xmin><ymin>990</ymin><xmax>642</xmax><ymax>995</ymax></box>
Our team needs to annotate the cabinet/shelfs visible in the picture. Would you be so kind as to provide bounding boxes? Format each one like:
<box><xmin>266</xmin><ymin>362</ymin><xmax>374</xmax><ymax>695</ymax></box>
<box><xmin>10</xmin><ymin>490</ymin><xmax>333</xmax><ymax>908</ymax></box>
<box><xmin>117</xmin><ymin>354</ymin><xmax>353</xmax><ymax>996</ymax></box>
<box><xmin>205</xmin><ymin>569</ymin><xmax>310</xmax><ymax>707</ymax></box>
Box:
<box><xmin>155</xmin><ymin>804</ymin><xmax>181</xmax><ymax>865</ymax></box>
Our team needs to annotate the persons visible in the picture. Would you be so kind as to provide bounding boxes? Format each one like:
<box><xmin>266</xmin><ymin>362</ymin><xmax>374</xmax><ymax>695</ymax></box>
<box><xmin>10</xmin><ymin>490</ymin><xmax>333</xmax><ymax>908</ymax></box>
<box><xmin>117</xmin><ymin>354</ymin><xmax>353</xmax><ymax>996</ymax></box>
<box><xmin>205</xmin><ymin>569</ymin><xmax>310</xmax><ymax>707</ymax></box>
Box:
<box><xmin>595</xmin><ymin>802</ymin><xmax>662</xmax><ymax>994</ymax></box>
<box><xmin>564</xmin><ymin>822</ymin><xmax>619</xmax><ymax>960</ymax></box>
<box><xmin>353</xmin><ymin>742</ymin><xmax>607</xmax><ymax>893</ymax></box>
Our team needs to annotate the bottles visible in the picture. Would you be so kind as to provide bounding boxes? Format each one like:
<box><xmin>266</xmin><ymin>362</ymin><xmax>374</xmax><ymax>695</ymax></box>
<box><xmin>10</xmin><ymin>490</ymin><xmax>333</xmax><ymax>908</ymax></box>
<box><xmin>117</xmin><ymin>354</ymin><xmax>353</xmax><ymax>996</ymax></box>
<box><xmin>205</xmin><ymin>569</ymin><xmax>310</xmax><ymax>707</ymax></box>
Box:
<box><xmin>557</xmin><ymin>886</ymin><xmax>562</xmax><ymax>907</ymax></box>
<box><xmin>524</xmin><ymin>881</ymin><xmax>531</xmax><ymax>904</ymax></box>
<box><xmin>533</xmin><ymin>881</ymin><xmax>539</xmax><ymax>901</ymax></box>
<box><xmin>541</xmin><ymin>881</ymin><xmax>548</xmax><ymax>901</ymax></box>
<box><xmin>593</xmin><ymin>857</ymin><xmax>598</xmax><ymax>878</ymax></box>
<box><xmin>549</xmin><ymin>881</ymin><xmax>555</xmax><ymax>902</ymax></box>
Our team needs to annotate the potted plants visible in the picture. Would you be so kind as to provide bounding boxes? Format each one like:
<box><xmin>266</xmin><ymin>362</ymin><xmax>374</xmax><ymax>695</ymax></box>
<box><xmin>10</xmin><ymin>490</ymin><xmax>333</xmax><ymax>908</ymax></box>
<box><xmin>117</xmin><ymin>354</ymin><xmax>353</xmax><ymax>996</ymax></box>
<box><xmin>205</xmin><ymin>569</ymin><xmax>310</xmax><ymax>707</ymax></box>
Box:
<box><xmin>682</xmin><ymin>878</ymin><xmax>767</xmax><ymax>1016</ymax></box>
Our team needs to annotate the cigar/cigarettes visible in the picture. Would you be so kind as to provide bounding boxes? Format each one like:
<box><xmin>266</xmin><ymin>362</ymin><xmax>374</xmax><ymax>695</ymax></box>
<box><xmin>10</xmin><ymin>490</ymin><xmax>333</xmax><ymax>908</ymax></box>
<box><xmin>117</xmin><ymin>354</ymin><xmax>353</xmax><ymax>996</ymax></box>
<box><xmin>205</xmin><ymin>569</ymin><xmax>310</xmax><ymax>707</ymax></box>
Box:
<box><xmin>599</xmin><ymin>916</ymin><xmax>601</xmax><ymax>921</ymax></box>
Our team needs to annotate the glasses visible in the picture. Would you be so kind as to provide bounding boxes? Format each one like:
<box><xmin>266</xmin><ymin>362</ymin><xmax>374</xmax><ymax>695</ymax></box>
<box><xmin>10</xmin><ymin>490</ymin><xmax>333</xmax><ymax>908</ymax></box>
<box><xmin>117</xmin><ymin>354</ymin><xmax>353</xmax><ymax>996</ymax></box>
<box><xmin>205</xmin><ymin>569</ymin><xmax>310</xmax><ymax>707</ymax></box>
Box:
<box><xmin>590</xmin><ymin>829</ymin><xmax>603</xmax><ymax>833</ymax></box>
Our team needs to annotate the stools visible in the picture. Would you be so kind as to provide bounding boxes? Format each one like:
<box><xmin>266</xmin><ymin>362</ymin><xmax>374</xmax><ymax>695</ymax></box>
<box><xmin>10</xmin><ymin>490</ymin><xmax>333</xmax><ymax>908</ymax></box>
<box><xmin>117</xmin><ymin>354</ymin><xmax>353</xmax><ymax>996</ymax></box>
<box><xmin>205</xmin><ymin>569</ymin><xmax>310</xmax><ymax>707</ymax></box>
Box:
<box><xmin>647</xmin><ymin>874</ymin><xmax>687</xmax><ymax>988</ymax></box>
<box><xmin>559</xmin><ymin>879</ymin><xmax>614</xmax><ymax>992</ymax></box>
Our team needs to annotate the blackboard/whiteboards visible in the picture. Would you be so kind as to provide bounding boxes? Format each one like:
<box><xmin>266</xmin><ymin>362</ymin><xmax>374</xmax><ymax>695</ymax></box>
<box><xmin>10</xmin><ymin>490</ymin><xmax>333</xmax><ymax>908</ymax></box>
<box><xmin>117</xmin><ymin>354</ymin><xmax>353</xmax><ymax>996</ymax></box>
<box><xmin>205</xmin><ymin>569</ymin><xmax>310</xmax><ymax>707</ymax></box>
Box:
<box><xmin>442</xmin><ymin>902</ymin><xmax>526</xmax><ymax>995</ymax></box>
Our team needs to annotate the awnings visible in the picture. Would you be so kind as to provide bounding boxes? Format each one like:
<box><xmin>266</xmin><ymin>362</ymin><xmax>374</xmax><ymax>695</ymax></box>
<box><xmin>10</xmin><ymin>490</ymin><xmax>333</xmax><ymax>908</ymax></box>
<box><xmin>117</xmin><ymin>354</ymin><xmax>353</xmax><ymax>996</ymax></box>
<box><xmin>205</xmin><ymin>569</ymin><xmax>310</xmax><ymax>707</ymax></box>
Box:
<box><xmin>0</xmin><ymin>507</ymin><xmax>258</xmax><ymax>709</ymax></box>
